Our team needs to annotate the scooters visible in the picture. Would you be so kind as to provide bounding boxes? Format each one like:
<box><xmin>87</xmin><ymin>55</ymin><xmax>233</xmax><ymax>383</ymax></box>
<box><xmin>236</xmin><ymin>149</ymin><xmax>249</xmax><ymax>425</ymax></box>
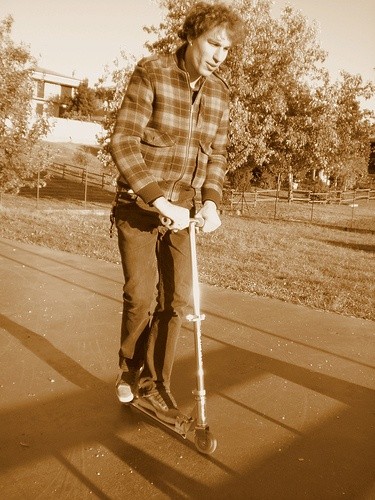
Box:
<box><xmin>116</xmin><ymin>218</ymin><xmax>219</xmax><ymax>455</ymax></box>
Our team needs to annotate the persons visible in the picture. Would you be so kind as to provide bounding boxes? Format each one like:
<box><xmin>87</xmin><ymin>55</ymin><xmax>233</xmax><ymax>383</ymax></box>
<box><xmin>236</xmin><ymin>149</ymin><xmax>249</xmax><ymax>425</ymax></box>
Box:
<box><xmin>107</xmin><ymin>4</ymin><xmax>243</xmax><ymax>427</ymax></box>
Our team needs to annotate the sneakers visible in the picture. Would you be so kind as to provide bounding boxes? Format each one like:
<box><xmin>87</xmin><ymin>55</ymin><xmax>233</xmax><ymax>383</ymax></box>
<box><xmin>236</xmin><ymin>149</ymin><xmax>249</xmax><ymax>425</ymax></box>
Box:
<box><xmin>116</xmin><ymin>370</ymin><xmax>139</xmax><ymax>403</ymax></box>
<box><xmin>137</xmin><ymin>384</ymin><xmax>184</xmax><ymax>425</ymax></box>
